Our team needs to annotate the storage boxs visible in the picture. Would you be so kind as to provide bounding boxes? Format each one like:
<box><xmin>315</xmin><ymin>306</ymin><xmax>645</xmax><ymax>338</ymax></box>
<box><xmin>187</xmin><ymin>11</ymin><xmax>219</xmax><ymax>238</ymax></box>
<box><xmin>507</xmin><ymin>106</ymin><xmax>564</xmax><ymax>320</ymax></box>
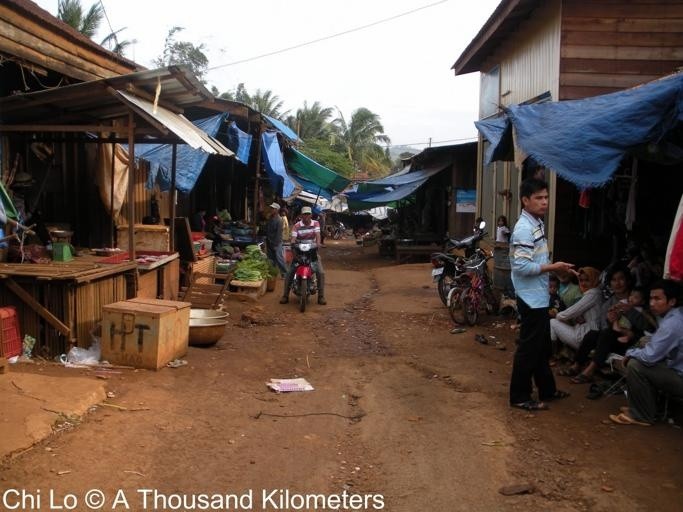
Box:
<box><xmin>101</xmin><ymin>297</ymin><xmax>192</xmax><ymax>372</ymax></box>
<box><xmin>229</xmin><ymin>279</ymin><xmax>267</xmax><ymax>297</ymax></box>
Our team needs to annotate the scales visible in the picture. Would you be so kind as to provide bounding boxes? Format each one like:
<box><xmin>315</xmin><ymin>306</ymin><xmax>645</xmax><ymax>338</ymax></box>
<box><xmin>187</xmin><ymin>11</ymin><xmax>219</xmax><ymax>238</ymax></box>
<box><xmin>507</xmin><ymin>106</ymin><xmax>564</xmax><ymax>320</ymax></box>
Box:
<box><xmin>50</xmin><ymin>230</ymin><xmax>73</xmax><ymax>263</ymax></box>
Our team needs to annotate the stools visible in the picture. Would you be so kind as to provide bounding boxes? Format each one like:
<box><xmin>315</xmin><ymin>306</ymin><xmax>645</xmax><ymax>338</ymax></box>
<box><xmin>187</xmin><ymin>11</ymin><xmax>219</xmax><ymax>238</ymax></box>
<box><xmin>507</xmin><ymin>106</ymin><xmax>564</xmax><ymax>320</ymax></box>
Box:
<box><xmin>603</xmin><ymin>361</ymin><xmax>630</xmax><ymax>395</ymax></box>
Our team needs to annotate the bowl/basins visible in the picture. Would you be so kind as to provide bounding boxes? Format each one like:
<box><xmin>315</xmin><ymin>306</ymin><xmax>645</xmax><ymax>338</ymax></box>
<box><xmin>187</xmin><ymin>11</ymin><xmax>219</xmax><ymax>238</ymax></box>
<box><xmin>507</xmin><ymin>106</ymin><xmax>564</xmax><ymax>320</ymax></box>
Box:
<box><xmin>187</xmin><ymin>319</ymin><xmax>228</xmax><ymax>347</ymax></box>
<box><xmin>189</xmin><ymin>309</ymin><xmax>230</xmax><ymax>319</ymax></box>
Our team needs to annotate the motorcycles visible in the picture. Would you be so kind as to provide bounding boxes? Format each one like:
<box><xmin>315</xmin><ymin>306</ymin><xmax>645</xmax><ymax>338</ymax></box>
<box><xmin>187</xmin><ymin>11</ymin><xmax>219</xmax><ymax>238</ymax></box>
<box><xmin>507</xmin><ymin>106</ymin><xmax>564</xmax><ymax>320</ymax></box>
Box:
<box><xmin>432</xmin><ymin>222</ymin><xmax>488</xmax><ymax>306</ymax></box>
<box><xmin>280</xmin><ymin>238</ymin><xmax>326</xmax><ymax>314</ymax></box>
<box><xmin>332</xmin><ymin>220</ymin><xmax>345</xmax><ymax>242</ymax></box>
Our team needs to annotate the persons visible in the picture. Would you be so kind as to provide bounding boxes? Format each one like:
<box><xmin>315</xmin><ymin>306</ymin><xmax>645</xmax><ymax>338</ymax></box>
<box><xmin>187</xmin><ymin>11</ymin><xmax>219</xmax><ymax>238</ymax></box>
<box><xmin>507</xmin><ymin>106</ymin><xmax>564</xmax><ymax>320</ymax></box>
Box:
<box><xmin>495</xmin><ymin>215</ymin><xmax>511</xmax><ymax>243</ymax></box>
<box><xmin>205</xmin><ymin>201</ymin><xmax>347</xmax><ymax>305</ymax></box>
<box><xmin>546</xmin><ymin>261</ymin><xmax>683</xmax><ymax>427</ymax></box>
<box><xmin>508</xmin><ymin>177</ymin><xmax>581</xmax><ymax>412</ymax></box>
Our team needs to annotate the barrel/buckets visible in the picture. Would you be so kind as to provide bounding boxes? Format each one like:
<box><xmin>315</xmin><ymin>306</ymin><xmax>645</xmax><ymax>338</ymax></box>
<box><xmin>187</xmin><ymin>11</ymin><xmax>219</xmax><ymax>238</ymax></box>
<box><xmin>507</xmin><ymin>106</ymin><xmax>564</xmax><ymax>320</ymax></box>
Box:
<box><xmin>494</xmin><ymin>246</ymin><xmax>511</xmax><ymax>288</ymax></box>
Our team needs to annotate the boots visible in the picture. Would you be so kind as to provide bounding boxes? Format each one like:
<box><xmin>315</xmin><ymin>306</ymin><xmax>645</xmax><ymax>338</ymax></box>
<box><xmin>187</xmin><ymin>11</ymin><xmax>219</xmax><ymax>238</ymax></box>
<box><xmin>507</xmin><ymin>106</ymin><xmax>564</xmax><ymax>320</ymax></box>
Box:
<box><xmin>278</xmin><ymin>276</ymin><xmax>293</xmax><ymax>304</ymax></box>
<box><xmin>316</xmin><ymin>272</ymin><xmax>327</xmax><ymax>305</ymax></box>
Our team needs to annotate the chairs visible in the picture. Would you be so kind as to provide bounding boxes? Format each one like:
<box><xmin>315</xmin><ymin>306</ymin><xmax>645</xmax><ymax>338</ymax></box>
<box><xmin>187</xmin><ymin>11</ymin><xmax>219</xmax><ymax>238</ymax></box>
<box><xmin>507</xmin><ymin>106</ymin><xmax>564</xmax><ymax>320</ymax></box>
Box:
<box><xmin>179</xmin><ymin>270</ymin><xmax>234</xmax><ymax>310</ymax></box>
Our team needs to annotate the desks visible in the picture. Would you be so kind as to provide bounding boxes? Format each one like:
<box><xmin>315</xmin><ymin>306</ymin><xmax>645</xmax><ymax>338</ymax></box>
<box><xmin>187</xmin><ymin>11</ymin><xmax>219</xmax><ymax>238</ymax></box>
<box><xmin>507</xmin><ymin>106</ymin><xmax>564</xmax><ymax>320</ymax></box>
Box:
<box><xmin>396</xmin><ymin>245</ymin><xmax>443</xmax><ymax>266</ymax></box>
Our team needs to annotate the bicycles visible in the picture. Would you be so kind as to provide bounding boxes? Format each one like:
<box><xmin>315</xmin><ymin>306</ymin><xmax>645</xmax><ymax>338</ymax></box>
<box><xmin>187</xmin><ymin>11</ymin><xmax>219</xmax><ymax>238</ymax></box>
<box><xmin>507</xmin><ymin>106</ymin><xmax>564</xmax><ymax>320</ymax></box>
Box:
<box><xmin>446</xmin><ymin>250</ymin><xmax>498</xmax><ymax>327</ymax></box>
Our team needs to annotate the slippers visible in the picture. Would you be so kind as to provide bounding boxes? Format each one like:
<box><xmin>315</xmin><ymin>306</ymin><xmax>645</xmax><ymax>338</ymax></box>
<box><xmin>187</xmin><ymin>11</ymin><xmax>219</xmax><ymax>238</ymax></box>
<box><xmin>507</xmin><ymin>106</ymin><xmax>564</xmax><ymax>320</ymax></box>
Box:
<box><xmin>609</xmin><ymin>413</ymin><xmax>652</xmax><ymax>427</ymax></box>
<box><xmin>585</xmin><ymin>382</ymin><xmax>603</xmax><ymax>401</ymax></box>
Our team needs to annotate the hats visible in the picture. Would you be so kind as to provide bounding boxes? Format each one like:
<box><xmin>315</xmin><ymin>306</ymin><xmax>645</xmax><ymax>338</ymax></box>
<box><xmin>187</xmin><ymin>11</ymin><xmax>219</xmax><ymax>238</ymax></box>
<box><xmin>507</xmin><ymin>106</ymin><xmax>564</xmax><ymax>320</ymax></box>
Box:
<box><xmin>301</xmin><ymin>206</ymin><xmax>312</xmax><ymax>215</ymax></box>
<box><xmin>269</xmin><ymin>202</ymin><xmax>280</xmax><ymax>210</ymax></box>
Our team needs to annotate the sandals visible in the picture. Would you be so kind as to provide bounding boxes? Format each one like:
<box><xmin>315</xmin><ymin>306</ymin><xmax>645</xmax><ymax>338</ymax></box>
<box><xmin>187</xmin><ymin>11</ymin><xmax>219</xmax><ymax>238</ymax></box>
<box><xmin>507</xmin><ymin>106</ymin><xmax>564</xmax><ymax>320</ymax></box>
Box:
<box><xmin>568</xmin><ymin>374</ymin><xmax>591</xmax><ymax>385</ymax></box>
<box><xmin>509</xmin><ymin>399</ymin><xmax>548</xmax><ymax>412</ymax></box>
<box><xmin>556</xmin><ymin>367</ymin><xmax>581</xmax><ymax>377</ymax></box>
<box><xmin>538</xmin><ymin>389</ymin><xmax>571</xmax><ymax>402</ymax></box>
